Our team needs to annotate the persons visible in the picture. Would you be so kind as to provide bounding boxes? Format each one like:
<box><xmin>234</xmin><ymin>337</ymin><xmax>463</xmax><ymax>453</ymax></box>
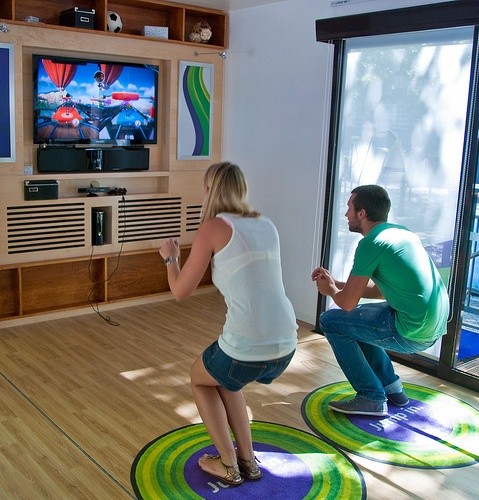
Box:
<box><xmin>311</xmin><ymin>184</ymin><xmax>449</xmax><ymax>416</ymax></box>
<box><xmin>160</xmin><ymin>161</ymin><xmax>298</xmax><ymax>485</ymax></box>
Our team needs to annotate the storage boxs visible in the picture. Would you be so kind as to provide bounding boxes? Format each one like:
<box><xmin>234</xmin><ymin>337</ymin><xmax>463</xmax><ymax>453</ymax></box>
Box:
<box><xmin>59</xmin><ymin>6</ymin><xmax>95</xmax><ymax>29</ymax></box>
<box><xmin>141</xmin><ymin>25</ymin><xmax>168</xmax><ymax>40</ymax></box>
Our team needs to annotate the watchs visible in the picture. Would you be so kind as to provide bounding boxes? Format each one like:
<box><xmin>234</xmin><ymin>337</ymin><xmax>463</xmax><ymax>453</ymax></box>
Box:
<box><xmin>164</xmin><ymin>256</ymin><xmax>178</xmax><ymax>265</ymax></box>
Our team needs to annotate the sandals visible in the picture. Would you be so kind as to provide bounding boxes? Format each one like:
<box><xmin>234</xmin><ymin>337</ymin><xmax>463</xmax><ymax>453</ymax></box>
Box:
<box><xmin>235</xmin><ymin>449</ymin><xmax>263</xmax><ymax>482</ymax></box>
<box><xmin>198</xmin><ymin>454</ymin><xmax>244</xmax><ymax>486</ymax></box>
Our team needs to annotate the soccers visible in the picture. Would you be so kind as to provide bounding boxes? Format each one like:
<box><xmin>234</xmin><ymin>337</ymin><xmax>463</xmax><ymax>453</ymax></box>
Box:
<box><xmin>107</xmin><ymin>10</ymin><xmax>122</xmax><ymax>33</ymax></box>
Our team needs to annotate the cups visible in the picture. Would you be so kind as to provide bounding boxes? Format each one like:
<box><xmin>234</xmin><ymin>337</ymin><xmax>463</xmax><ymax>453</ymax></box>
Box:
<box><xmin>24</xmin><ymin>164</ymin><xmax>33</xmax><ymax>174</ymax></box>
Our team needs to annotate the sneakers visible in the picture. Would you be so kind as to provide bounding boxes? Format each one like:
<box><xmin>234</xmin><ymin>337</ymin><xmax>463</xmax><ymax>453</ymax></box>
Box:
<box><xmin>387</xmin><ymin>390</ymin><xmax>409</xmax><ymax>406</ymax></box>
<box><xmin>328</xmin><ymin>397</ymin><xmax>389</xmax><ymax>416</ymax></box>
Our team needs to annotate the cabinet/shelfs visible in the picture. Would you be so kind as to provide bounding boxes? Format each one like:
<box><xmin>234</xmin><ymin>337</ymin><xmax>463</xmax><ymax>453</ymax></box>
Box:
<box><xmin>0</xmin><ymin>0</ymin><xmax>230</xmax><ymax>50</ymax></box>
<box><xmin>1</xmin><ymin>243</ymin><xmax>214</xmax><ymax>321</ymax></box>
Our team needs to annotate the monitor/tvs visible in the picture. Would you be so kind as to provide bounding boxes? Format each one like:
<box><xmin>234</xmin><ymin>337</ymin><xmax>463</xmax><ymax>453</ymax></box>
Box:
<box><xmin>29</xmin><ymin>55</ymin><xmax>160</xmax><ymax>148</ymax></box>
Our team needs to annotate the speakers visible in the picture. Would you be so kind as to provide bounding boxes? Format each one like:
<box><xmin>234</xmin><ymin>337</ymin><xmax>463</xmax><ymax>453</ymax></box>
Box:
<box><xmin>102</xmin><ymin>146</ymin><xmax>148</xmax><ymax>171</ymax></box>
<box><xmin>35</xmin><ymin>147</ymin><xmax>89</xmax><ymax>173</ymax></box>
<box><xmin>91</xmin><ymin>211</ymin><xmax>105</xmax><ymax>245</ymax></box>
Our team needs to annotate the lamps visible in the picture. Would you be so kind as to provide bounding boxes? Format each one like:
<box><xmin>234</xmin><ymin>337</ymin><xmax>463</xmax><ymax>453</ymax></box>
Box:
<box><xmin>193</xmin><ymin>50</ymin><xmax>228</xmax><ymax>58</ymax></box>
<box><xmin>0</xmin><ymin>24</ymin><xmax>10</xmax><ymax>33</ymax></box>
